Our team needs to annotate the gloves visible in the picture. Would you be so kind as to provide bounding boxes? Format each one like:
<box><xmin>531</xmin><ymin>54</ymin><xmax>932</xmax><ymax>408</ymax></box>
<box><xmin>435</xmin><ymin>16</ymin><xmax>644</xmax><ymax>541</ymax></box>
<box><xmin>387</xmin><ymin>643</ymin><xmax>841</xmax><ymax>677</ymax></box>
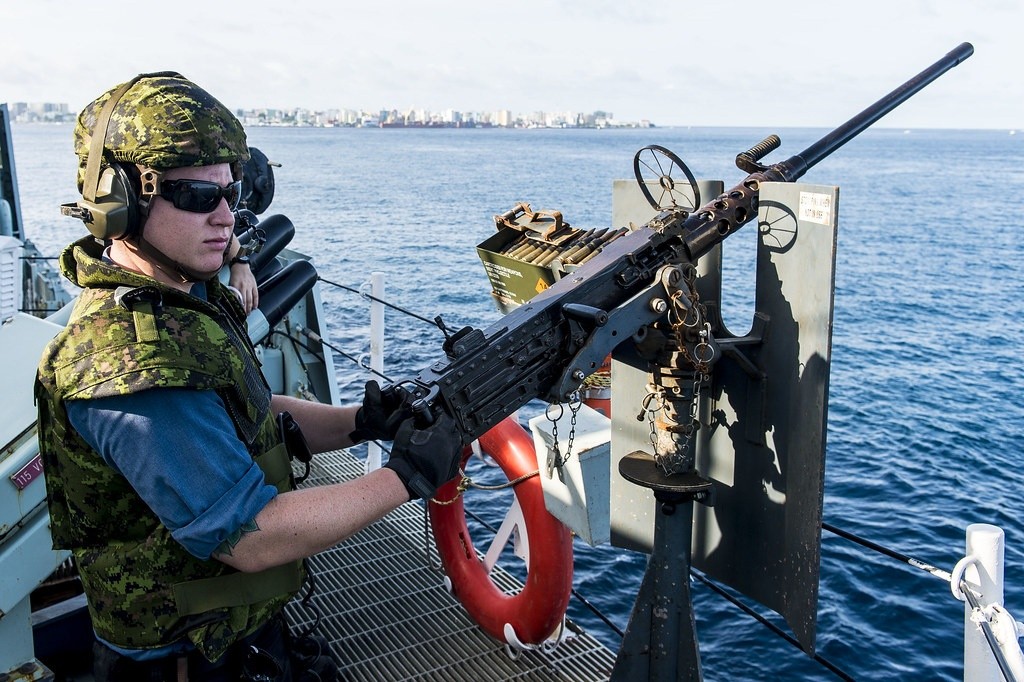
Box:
<box><xmin>349</xmin><ymin>380</ymin><xmax>417</xmax><ymax>444</ymax></box>
<box><xmin>382</xmin><ymin>406</ymin><xmax>462</xmax><ymax>502</ymax></box>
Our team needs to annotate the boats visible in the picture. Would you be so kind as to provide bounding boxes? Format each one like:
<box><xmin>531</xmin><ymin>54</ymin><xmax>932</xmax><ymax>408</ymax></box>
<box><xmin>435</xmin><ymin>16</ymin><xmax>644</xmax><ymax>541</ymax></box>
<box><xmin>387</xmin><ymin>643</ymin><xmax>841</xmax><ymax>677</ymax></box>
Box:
<box><xmin>0</xmin><ymin>100</ymin><xmax>1024</xmax><ymax>682</ymax></box>
<box><xmin>474</xmin><ymin>124</ymin><xmax>483</xmax><ymax>129</ymax></box>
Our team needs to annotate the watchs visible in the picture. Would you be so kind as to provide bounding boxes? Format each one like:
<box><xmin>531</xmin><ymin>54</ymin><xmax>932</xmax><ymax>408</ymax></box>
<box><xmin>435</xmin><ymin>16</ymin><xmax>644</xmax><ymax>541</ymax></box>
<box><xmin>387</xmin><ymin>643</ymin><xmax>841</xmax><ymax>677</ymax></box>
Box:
<box><xmin>229</xmin><ymin>256</ymin><xmax>252</xmax><ymax>271</ymax></box>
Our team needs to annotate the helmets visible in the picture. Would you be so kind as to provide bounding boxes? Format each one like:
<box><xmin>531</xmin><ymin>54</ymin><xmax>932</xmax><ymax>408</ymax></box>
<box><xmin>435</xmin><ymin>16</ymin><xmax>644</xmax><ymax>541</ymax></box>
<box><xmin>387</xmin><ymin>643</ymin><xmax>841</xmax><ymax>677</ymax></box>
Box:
<box><xmin>74</xmin><ymin>71</ymin><xmax>251</xmax><ymax>195</ymax></box>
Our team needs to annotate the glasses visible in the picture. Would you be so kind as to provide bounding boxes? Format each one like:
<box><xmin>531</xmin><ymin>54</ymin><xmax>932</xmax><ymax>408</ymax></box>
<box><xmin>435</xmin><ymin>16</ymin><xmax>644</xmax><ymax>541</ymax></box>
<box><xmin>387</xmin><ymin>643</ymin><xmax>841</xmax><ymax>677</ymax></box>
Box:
<box><xmin>158</xmin><ymin>178</ymin><xmax>242</xmax><ymax>214</ymax></box>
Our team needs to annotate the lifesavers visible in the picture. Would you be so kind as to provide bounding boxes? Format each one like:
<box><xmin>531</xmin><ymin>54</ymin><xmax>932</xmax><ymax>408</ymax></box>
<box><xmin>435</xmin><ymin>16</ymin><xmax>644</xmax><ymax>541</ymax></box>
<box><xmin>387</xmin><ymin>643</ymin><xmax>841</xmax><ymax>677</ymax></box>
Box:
<box><xmin>426</xmin><ymin>413</ymin><xmax>576</xmax><ymax>648</ymax></box>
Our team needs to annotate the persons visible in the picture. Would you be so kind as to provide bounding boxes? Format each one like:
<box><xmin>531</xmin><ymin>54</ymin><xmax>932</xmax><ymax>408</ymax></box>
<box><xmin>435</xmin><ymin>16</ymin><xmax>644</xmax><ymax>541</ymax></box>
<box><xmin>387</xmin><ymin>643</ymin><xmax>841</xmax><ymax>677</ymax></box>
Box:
<box><xmin>34</xmin><ymin>71</ymin><xmax>463</xmax><ymax>682</ymax></box>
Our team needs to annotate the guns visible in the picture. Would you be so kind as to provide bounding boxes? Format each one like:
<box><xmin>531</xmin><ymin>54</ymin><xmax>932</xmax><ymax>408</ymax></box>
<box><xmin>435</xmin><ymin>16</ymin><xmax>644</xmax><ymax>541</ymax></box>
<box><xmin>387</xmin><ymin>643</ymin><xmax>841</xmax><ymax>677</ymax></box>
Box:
<box><xmin>362</xmin><ymin>38</ymin><xmax>978</xmax><ymax>485</ymax></box>
